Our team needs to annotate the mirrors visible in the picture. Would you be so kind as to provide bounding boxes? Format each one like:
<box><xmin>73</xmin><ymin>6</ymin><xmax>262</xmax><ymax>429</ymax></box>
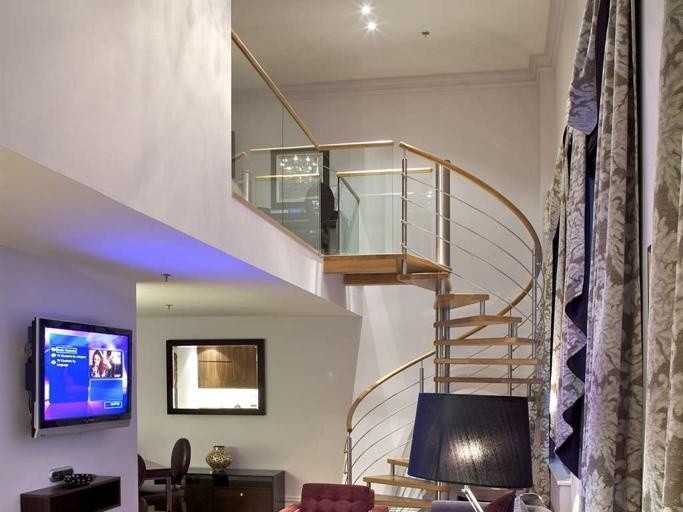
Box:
<box><xmin>165</xmin><ymin>338</ymin><xmax>265</xmax><ymax>416</ymax></box>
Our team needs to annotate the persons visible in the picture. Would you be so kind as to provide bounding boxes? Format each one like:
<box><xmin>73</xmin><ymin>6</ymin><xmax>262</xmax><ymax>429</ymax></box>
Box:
<box><xmin>105</xmin><ymin>351</ymin><xmax>114</xmax><ymax>377</ymax></box>
<box><xmin>90</xmin><ymin>350</ymin><xmax>111</xmax><ymax>377</ymax></box>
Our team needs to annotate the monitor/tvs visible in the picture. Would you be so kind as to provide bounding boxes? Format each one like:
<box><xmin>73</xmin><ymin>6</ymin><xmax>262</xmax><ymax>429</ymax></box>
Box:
<box><xmin>25</xmin><ymin>316</ymin><xmax>132</xmax><ymax>439</ymax></box>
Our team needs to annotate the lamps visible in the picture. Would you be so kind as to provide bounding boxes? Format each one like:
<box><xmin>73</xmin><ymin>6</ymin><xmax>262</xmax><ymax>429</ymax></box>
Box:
<box><xmin>407</xmin><ymin>391</ymin><xmax>534</xmax><ymax>512</ymax></box>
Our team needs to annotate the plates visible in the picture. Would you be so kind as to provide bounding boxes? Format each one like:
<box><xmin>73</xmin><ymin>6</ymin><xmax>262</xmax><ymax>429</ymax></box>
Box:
<box><xmin>63</xmin><ymin>473</ymin><xmax>97</xmax><ymax>486</ymax></box>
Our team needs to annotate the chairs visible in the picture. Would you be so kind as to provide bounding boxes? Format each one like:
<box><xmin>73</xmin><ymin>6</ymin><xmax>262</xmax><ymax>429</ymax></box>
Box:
<box><xmin>276</xmin><ymin>481</ymin><xmax>389</xmax><ymax>511</ymax></box>
<box><xmin>137</xmin><ymin>435</ymin><xmax>191</xmax><ymax>512</ymax></box>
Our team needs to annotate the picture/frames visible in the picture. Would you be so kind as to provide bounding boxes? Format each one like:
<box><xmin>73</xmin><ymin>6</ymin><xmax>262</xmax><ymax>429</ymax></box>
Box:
<box><xmin>269</xmin><ymin>147</ymin><xmax>329</xmax><ymax>211</ymax></box>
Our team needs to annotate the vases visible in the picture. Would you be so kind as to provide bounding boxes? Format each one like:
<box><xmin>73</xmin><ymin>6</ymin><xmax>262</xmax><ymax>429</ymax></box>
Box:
<box><xmin>204</xmin><ymin>444</ymin><xmax>232</xmax><ymax>470</ymax></box>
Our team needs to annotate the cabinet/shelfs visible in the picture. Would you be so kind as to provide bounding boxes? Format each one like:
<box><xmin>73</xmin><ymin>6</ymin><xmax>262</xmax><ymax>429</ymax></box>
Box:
<box><xmin>186</xmin><ymin>468</ymin><xmax>286</xmax><ymax>511</ymax></box>
